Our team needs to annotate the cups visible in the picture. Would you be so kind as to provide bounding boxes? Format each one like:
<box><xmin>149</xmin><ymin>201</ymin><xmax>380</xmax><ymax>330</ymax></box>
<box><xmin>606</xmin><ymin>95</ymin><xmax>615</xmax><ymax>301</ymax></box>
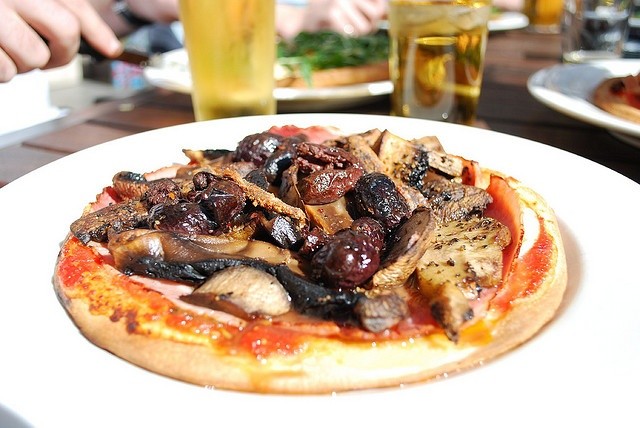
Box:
<box><xmin>175</xmin><ymin>1</ymin><xmax>276</xmax><ymax>122</ymax></box>
<box><xmin>525</xmin><ymin>0</ymin><xmax>565</xmax><ymax>35</ymax></box>
<box><xmin>384</xmin><ymin>2</ymin><xmax>490</xmax><ymax>127</ymax></box>
<box><xmin>560</xmin><ymin>2</ymin><xmax>631</xmax><ymax>62</ymax></box>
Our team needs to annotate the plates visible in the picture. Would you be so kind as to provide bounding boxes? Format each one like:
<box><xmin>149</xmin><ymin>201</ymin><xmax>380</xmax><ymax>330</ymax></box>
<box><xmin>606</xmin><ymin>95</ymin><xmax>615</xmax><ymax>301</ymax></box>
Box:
<box><xmin>1</xmin><ymin>113</ymin><xmax>639</xmax><ymax>428</ymax></box>
<box><xmin>143</xmin><ymin>46</ymin><xmax>396</xmax><ymax>112</ymax></box>
<box><xmin>489</xmin><ymin>10</ymin><xmax>530</xmax><ymax>34</ymax></box>
<box><xmin>526</xmin><ymin>57</ymin><xmax>640</xmax><ymax>147</ymax></box>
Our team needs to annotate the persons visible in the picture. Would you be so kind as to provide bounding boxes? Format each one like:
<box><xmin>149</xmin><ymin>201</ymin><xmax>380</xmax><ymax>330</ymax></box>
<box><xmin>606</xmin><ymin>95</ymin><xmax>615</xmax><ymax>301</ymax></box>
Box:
<box><xmin>1</xmin><ymin>0</ymin><xmax>124</xmax><ymax>82</ymax></box>
<box><xmin>128</xmin><ymin>1</ymin><xmax>387</xmax><ymax>42</ymax></box>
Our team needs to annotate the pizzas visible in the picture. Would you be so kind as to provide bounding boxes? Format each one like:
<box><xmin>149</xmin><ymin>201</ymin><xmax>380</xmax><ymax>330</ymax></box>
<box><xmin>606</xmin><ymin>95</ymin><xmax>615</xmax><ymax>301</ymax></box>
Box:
<box><xmin>592</xmin><ymin>69</ymin><xmax>640</xmax><ymax>124</ymax></box>
<box><xmin>50</xmin><ymin>123</ymin><xmax>569</xmax><ymax>398</ymax></box>
<box><xmin>273</xmin><ymin>60</ymin><xmax>393</xmax><ymax>87</ymax></box>
